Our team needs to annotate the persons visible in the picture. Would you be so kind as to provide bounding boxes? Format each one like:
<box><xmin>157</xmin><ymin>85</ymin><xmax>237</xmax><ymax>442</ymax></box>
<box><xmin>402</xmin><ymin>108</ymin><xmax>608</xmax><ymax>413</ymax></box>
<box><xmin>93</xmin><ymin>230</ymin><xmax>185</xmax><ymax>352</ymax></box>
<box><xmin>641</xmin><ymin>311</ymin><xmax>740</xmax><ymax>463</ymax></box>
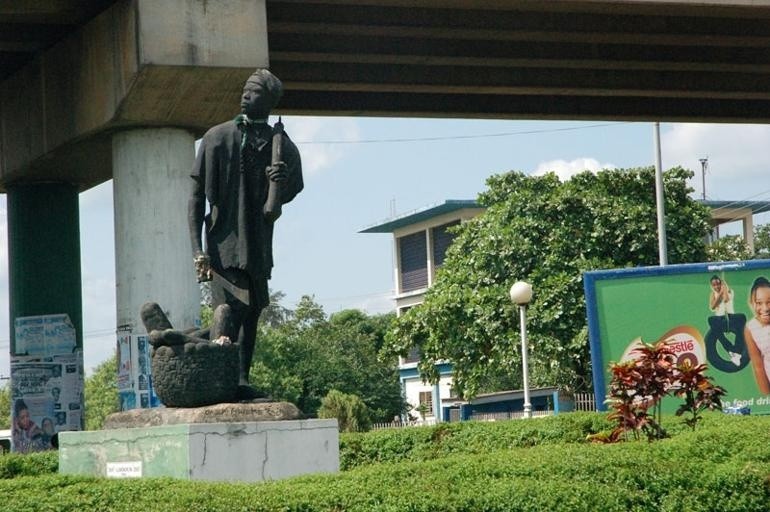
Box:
<box><xmin>33</xmin><ymin>417</ymin><xmax>59</xmax><ymax>452</ymax></box>
<box><xmin>11</xmin><ymin>398</ymin><xmax>41</xmax><ymax>453</ymax></box>
<box><xmin>52</xmin><ymin>387</ymin><xmax>61</xmax><ymax>403</ymax></box>
<box><xmin>707</xmin><ymin>274</ymin><xmax>747</xmax><ymax>368</ymax></box>
<box><xmin>742</xmin><ymin>275</ymin><xmax>770</xmax><ymax>395</ymax></box>
<box><xmin>47</xmin><ymin>365</ymin><xmax>62</xmax><ymax>384</ymax></box>
<box><xmin>184</xmin><ymin>66</ymin><xmax>306</xmax><ymax>402</ymax></box>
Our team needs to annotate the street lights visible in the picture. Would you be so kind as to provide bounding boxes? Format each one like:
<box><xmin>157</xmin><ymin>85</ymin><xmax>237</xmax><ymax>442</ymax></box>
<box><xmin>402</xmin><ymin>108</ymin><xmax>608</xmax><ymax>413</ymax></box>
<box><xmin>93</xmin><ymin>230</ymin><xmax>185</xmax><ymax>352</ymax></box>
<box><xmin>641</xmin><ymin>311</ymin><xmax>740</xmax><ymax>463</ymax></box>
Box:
<box><xmin>698</xmin><ymin>152</ymin><xmax>711</xmax><ymax>199</ymax></box>
<box><xmin>509</xmin><ymin>279</ymin><xmax>533</xmax><ymax>419</ymax></box>
